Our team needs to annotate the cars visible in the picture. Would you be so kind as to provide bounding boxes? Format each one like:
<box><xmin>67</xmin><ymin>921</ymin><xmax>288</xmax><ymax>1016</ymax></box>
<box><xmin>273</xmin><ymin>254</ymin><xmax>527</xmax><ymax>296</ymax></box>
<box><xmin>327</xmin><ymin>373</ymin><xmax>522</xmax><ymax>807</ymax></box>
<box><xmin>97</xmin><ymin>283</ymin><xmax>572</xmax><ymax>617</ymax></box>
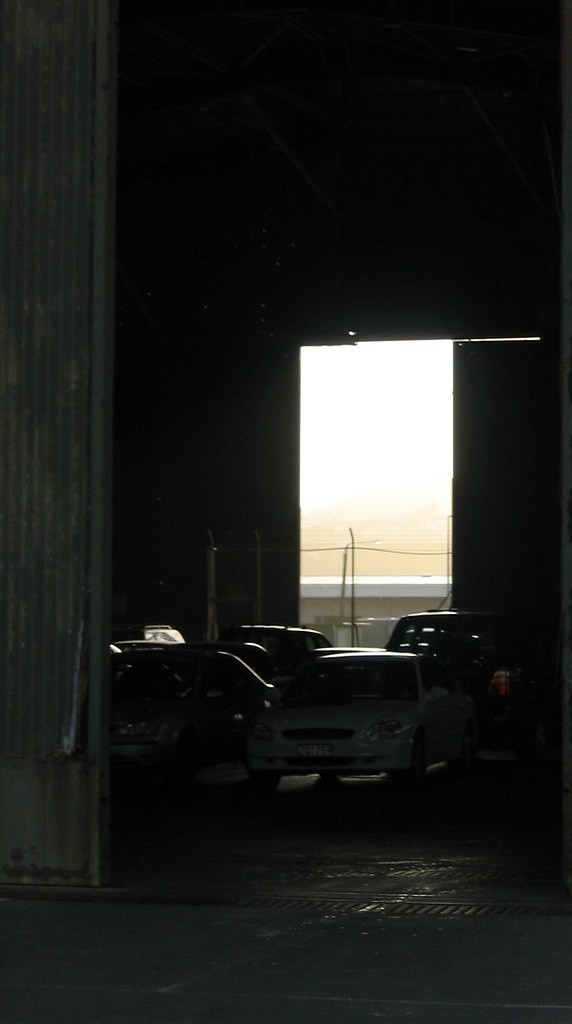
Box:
<box><xmin>105</xmin><ymin>640</ymin><xmax>283</xmax><ymax>781</ymax></box>
<box><xmin>217</xmin><ymin>624</ymin><xmax>332</xmax><ymax>648</ymax></box>
<box><xmin>384</xmin><ymin>608</ymin><xmax>524</xmax><ymax>716</ymax></box>
<box><xmin>244</xmin><ymin>651</ymin><xmax>481</xmax><ymax>796</ymax></box>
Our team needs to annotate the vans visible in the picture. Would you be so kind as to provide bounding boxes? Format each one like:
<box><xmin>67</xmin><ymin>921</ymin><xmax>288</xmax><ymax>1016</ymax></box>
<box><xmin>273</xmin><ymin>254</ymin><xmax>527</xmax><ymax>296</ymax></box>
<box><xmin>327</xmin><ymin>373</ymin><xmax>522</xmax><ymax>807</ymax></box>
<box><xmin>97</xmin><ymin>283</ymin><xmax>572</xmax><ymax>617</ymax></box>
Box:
<box><xmin>110</xmin><ymin>623</ymin><xmax>187</xmax><ymax>645</ymax></box>
